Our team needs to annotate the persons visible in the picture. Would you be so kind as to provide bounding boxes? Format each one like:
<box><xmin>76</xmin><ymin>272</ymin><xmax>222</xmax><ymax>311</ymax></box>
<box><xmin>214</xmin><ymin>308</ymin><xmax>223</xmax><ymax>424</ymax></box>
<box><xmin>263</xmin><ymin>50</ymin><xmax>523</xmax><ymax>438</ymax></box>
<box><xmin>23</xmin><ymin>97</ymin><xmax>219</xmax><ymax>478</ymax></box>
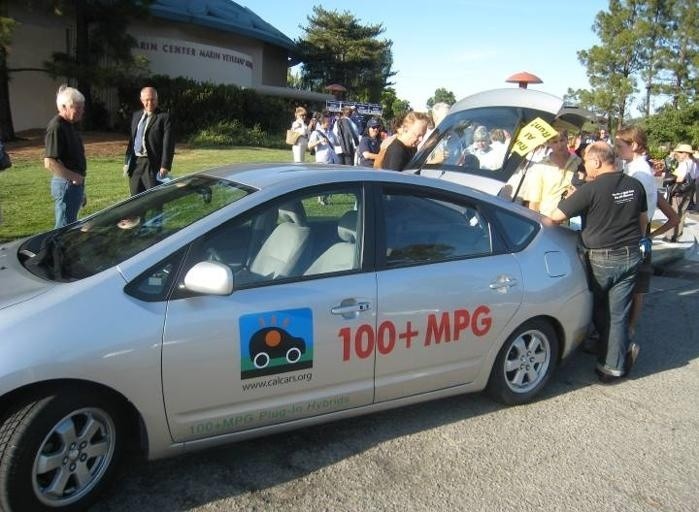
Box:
<box><xmin>290</xmin><ymin>106</ymin><xmax>386</xmax><ymax>204</ymax></box>
<box><xmin>549</xmin><ymin>140</ymin><xmax>649</xmax><ymax>382</ymax></box>
<box><xmin>513</xmin><ymin>126</ymin><xmax>697</xmax><ymax>243</ymax></box>
<box><xmin>120</xmin><ymin>87</ymin><xmax>175</xmax><ymax>234</ymax></box>
<box><xmin>42</xmin><ymin>82</ymin><xmax>89</xmax><ymax>231</ymax></box>
<box><xmin>381</xmin><ymin>101</ymin><xmax>511</xmax><ymax>173</ymax></box>
<box><xmin>611</xmin><ymin>126</ymin><xmax>659</xmax><ymax>365</ymax></box>
<box><xmin>117</xmin><ymin>215</ymin><xmax>141</xmax><ymax>232</ymax></box>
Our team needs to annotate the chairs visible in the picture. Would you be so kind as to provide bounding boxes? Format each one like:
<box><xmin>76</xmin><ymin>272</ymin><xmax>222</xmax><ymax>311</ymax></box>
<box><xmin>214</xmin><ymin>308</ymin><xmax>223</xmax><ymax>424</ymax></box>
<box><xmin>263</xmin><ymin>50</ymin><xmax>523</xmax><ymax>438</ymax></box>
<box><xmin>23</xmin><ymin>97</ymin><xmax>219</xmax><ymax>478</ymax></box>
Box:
<box><xmin>234</xmin><ymin>201</ymin><xmax>312</xmax><ymax>287</ymax></box>
<box><xmin>302</xmin><ymin>210</ymin><xmax>360</xmax><ymax>277</ymax></box>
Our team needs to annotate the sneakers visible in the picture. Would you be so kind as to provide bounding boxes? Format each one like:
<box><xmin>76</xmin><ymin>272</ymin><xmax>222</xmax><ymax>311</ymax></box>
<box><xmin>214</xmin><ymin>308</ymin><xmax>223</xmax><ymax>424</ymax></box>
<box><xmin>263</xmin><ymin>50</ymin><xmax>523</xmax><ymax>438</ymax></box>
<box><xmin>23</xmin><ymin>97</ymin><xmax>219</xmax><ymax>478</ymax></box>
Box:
<box><xmin>319</xmin><ymin>197</ymin><xmax>329</xmax><ymax>206</ymax></box>
<box><xmin>623</xmin><ymin>343</ymin><xmax>640</xmax><ymax>376</ymax></box>
<box><xmin>594</xmin><ymin>364</ymin><xmax>613</xmax><ymax>383</ymax></box>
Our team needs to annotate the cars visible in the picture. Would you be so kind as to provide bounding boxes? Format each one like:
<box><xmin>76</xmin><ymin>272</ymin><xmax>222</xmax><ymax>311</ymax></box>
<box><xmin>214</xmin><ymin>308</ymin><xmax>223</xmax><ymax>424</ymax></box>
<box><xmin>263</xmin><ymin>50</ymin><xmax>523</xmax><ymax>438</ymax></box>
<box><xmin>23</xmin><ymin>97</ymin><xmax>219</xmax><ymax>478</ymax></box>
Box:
<box><xmin>0</xmin><ymin>88</ymin><xmax>597</xmax><ymax>510</ymax></box>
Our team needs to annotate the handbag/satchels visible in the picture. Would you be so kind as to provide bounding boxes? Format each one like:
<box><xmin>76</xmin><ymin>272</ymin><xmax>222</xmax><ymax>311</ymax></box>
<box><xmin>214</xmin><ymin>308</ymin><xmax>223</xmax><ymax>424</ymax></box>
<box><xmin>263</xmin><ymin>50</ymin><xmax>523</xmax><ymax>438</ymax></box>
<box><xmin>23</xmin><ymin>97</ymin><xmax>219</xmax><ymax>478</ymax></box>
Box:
<box><xmin>286</xmin><ymin>129</ymin><xmax>300</xmax><ymax>145</ymax></box>
<box><xmin>673</xmin><ymin>174</ymin><xmax>695</xmax><ymax>194</ymax></box>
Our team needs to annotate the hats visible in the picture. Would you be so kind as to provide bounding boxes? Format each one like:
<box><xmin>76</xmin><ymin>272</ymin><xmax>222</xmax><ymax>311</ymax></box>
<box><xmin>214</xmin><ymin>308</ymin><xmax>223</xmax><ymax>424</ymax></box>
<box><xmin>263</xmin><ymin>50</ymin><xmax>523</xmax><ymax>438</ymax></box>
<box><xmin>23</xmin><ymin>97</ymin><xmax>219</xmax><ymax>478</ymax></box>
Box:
<box><xmin>674</xmin><ymin>144</ymin><xmax>696</xmax><ymax>154</ymax></box>
<box><xmin>367</xmin><ymin>119</ymin><xmax>381</xmax><ymax>128</ymax></box>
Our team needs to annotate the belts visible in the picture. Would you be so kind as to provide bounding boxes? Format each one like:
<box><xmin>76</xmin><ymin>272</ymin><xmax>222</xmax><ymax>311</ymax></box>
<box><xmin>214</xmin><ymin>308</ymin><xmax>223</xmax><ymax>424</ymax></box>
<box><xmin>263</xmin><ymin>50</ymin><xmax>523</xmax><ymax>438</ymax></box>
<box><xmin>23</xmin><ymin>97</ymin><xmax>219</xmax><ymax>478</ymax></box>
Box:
<box><xmin>592</xmin><ymin>247</ymin><xmax>638</xmax><ymax>256</ymax></box>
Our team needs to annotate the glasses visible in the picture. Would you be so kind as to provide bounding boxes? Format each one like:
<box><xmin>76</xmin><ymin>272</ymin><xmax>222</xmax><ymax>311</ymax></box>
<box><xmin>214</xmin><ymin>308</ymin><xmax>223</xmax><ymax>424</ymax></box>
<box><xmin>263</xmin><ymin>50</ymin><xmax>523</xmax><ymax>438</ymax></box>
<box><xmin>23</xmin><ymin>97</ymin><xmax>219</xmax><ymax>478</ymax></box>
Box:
<box><xmin>372</xmin><ymin>126</ymin><xmax>379</xmax><ymax>129</ymax></box>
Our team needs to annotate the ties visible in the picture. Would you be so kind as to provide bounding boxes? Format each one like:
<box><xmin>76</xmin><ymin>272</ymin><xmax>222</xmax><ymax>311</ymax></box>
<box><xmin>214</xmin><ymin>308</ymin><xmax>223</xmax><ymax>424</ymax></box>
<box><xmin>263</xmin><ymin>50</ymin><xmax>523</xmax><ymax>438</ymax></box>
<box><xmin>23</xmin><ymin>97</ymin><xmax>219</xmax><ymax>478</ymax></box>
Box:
<box><xmin>134</xmin><ymin>113</ymin><xmax>148</xmax><ymax>153</ymax></box>
